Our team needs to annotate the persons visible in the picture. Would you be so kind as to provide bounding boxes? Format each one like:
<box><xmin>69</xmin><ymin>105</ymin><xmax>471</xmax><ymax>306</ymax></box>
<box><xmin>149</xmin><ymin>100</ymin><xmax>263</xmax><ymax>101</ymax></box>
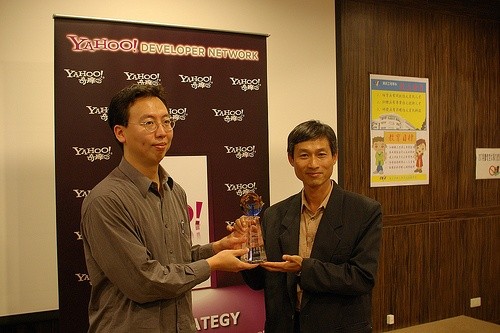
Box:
<box><xmin>227</xmin><ymin>120</ymin><xmax>382</xmax><ymax>333</ymax></box>
<box><xmin>80</xmin><ymin>84</ymin><xmax>259</xmax><ymax>332</ymax></box>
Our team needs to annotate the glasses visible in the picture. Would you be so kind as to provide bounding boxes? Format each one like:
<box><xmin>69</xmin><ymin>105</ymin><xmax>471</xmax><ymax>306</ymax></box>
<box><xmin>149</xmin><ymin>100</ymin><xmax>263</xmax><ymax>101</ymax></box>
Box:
<box><xmin>121</xmin><ymin>116</ymin><xmax>176</xmax><ymax>133</ymax></box>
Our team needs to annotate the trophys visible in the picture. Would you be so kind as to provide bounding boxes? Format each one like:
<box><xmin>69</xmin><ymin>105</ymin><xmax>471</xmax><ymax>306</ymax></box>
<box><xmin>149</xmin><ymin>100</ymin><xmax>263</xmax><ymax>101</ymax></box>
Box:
<box><xmin>238</xmin><ymin>191</ymin><xmax>267</xmax><ymax>264</ymax></box>
